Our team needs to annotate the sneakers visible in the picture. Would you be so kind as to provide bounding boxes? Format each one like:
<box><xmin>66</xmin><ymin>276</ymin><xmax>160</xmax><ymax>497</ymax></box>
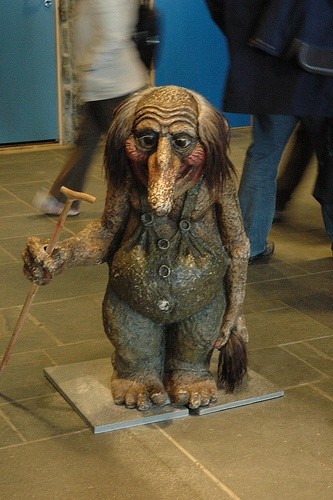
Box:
<box><xmin>41</xmin><ymin>199</ymin><xmax>80</xmax><ymax>216</ymax></box>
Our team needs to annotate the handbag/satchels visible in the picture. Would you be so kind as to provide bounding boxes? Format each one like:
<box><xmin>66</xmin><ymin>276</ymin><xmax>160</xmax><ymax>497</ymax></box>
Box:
<box><xmin>133</xmin><ymin>4</ymin><xmax>158</xmax><ymax>65</ymax></box>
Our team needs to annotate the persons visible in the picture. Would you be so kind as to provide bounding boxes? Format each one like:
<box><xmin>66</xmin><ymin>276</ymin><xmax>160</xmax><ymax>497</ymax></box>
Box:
<box><xmin>205</xmin><ymin>0</ymin><xmax>333</xmax><ymax>265</ymax></box>
<box><xmin>32</xmin><ymin>0</ymin><xmax>151</xmax><ymax>218</ymax></box>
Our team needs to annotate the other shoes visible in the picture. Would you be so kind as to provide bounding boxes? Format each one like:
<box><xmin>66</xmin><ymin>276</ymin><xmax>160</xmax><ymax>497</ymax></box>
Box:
<box><xmin>246</xmin><ymin>239</ymin><xmax>274</xmax><ymax>263</ymax></box>
